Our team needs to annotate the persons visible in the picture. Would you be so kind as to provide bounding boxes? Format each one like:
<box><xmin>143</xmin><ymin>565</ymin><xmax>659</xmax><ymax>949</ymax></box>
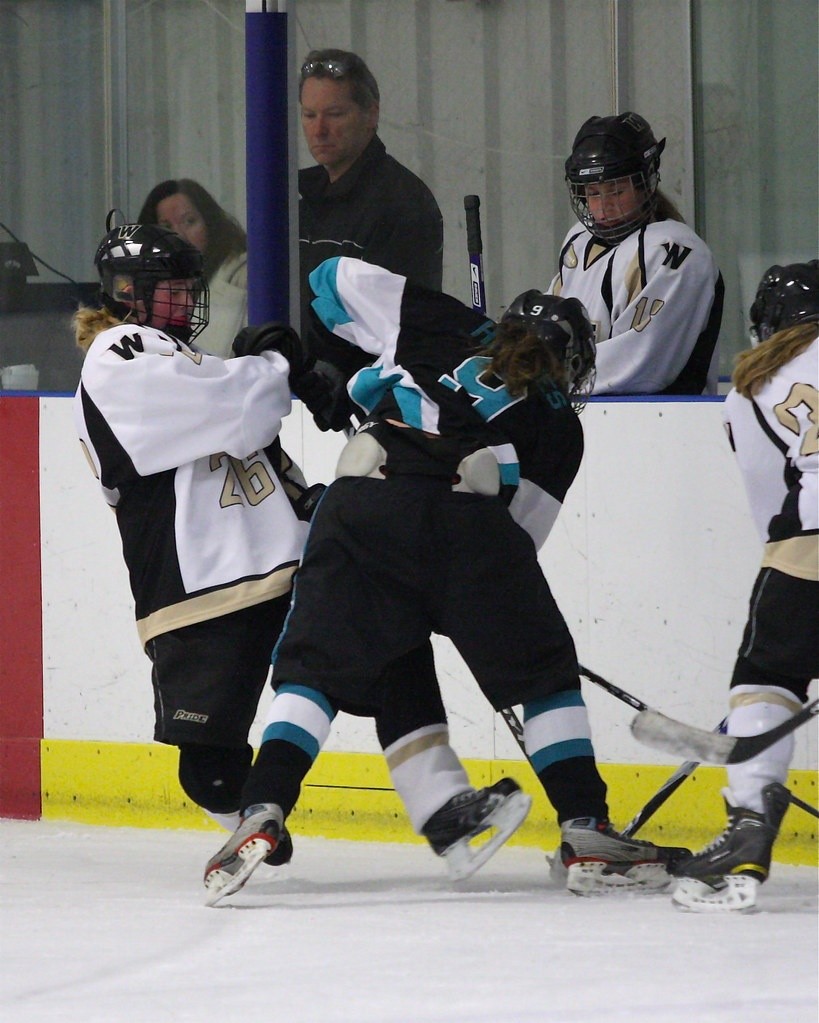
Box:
<box><xmin>666</xmin><ymin>259</ymin><xmax>819</xmax><ymax>883</ymax></box>
<box><xmin>72</xmin><ymin>48</ymin><xmax>728</xmax><ymax>865</ymax></box>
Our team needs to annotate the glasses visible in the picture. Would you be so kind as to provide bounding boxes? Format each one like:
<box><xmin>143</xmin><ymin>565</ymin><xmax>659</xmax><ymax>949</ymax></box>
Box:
<box><xmin>301</xmin><ymin>60</ymin><xmax>377</xmax><ymax>104</ymax></box>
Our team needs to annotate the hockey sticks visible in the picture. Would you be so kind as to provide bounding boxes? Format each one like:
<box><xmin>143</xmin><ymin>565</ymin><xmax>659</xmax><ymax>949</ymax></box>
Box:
<box><xmin>341</xmin><ymin>419</ymin><xmax>531</xmax><ymax>766</ymax></box>
<box><xmin>458</xmin><ymin>191</ymin><xmax>489</xmax><ymax>317</ymax></box>
<box><xmin>630</xmin><ymin>699</ymin><xmax>818</xmax><ymax>766</ymax></box>
<box><xmin>280</xmin><ymin>478</ymin><xmax>819</xmax><ymax>821</ymax></box>
<box><xmin>618</xmin><ymin>714</ymin><xmax>730</xmax><ymax>841</ymax></box>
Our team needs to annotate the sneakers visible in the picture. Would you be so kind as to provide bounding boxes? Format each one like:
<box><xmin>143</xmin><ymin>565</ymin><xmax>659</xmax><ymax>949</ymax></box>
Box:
<box><xmin>561</xmin><ymin>814</ymin><xmax>697</xmax><ymax>897</ymax></box>
<box><xmin>200</xmin><ymin>805</ymin><xmax>282</xmax><ymax>907</ymax></box>
<box><xmin>420</xmin><ymin>776</ymin><xmax>533</xmax><ymax>882</ymax></box>
<box><xmin>671</xmin><ymin>781</ymin><xmax>795</xmax><ymax>911</ymax></box>
<box><xmin>209</xmin><ymin>800</ymin><xmax>293</xmax><ymax>866</ymax></box>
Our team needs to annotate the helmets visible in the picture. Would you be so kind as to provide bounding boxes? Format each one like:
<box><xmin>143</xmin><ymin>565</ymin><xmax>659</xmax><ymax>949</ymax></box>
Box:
<box><xmin>562</xmin><ymin>111</ymin><xmax>666</xmax><ymax>244</ymax></box>
<box><xmin>500</xmin><ymin>289</ymin><xmax>596</xmax><ymax>416</ymax></box>
<box><xmin>749</xmin><ymin>259</ymin><xmax>819</xmax><ymax>341</ymax></box>
<box><xmin>94</xmin><ymin>223</ymin><xmax>209</xmax><ymax>346</ymax></box>
